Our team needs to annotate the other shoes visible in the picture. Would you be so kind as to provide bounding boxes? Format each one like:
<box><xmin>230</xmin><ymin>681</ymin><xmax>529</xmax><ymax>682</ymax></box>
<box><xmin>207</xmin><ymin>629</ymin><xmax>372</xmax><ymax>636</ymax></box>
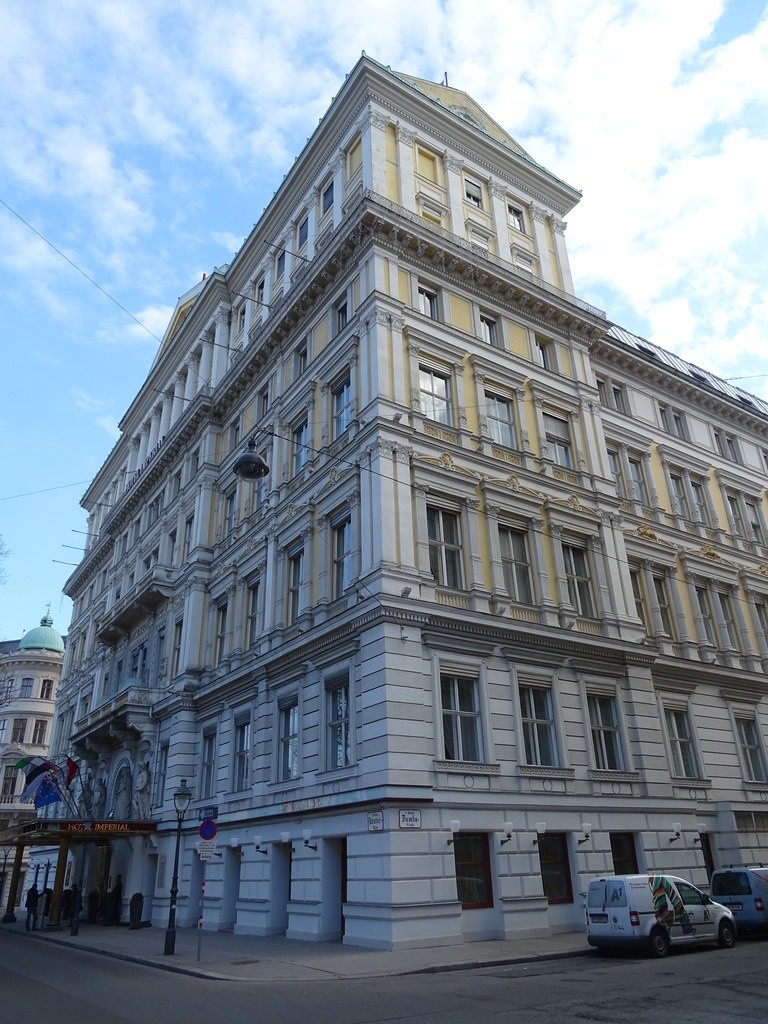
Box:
<box><xmin>26</xmin><ymin>928</ymin><xmax>29</xmax><ymax>931</ymax></box>
<box><xmin>32</xmin><ymin>927</ymin><xmax>38</xmax><ymax>930</ymax></box>
<box><xmin>68</xmin><ymin>923</ymin><xmax>72</xmax><ymax>927</ymax></box>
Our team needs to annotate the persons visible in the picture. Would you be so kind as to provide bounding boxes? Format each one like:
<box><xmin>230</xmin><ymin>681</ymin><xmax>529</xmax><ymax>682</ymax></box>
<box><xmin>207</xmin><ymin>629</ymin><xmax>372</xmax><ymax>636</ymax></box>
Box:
<box><xmin>92</xmin><ymin>778</ymin><xmax>105</xmax><ymax>817</ymax></box>
<box><xmin>133</xmin><ymin>760</ymin><xmax>151</xmax><ymax>818</ymax></box>
<box><xmin>79</xmin><ymin>784</ymin><xmax>93</xmax><ymax>817</ymax></box>
<box><xmin>70</xmin><ymin>883</ymin><xmax>84</xmax><ymax>936</ymax></box>
<box><xmin>25</xmin><ymin>884</ymin><xmax>44</xmax><ymax>931</ymax></box>
<box><xmin>115</xmin><ymin>767</ymin><xmax>130</xmax><ymax>819</ymax></box>
<box><xmin>108</xmin><ymin>874</ymin><xmax>124</xmax><ymax>925</ymax></box>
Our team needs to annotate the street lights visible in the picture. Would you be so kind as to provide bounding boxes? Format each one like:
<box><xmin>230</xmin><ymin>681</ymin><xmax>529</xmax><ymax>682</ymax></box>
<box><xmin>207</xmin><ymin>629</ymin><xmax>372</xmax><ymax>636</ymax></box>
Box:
<box><xmin>164</xmin><ymin>778</ymin><xmax>193</xmax><ymax>955</ymax></box>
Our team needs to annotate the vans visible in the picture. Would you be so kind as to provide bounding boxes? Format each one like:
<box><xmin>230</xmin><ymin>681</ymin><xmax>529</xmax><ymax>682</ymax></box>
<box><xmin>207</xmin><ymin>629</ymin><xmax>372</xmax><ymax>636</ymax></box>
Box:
<box><xmin>585</xmin><ymin>875</ymin><xmax>738</xmax><ymax>956</ymax></box>
<box><xmin>709</xmin><ymin>862</ymin><xmax>768</xmax><ymax>940</ymax></box>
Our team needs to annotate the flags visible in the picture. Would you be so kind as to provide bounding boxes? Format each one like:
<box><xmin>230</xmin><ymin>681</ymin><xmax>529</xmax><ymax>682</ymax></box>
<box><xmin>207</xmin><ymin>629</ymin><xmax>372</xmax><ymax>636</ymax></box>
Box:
<box><xmin>14</xmin><ymin>754</ymin><xmax>79</xmax><ymax>810</ymax></box>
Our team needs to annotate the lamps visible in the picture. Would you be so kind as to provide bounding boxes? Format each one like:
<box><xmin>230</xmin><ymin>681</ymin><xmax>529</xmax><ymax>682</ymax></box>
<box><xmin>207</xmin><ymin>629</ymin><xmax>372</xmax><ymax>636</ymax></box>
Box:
<box><xmin>695</xmin><ymin>823</ymin><xmax>707</xmax><ymax>844</ymax></box>
<box><xmin>447</xmin><ymin>820</ymin><xmax>461</xmax><ymax>845</ymax></box>
<box><xmin>578</xmin><ymin>823</ymin><xmax>592</xmax><ymax>845</ymax></box>
<box><xmin>708</xmin><ymin>658</ymin><xmax>717</xmax><ymax>664</ymax></box>
<box><xmin>669</xmin><ymin>823</ymin><xmax>682</xmax><ymax>842</ymax></box>
<box><xmin>400</xmin><ymin>586</ymin><xmax>412</xmax><ymax>597</ymax></box>
<box><xmin>232</xmin><ymin>430</ymin><xmax>270</xmax><ymax>480</ymax></box>
<box><xmin>533</xmin><ymin>822</ymin><xmax>546</xmax><ymax>845</ymax></box>
<box><xmin>254</xmin><ymin>836</ymin><xmax>268</xmax><ymax>855</ymax></box>
<box><xmin>565</xmin><ymin>621</ymin><xmax>575</xmax><ymax>629</ymax></box>
<box><xmin>495</xmin><ymin>606</ymin><xmax>506</xmax><ymax>616</ymax></box>
<box><xmin>302</xmin><ymin>829</ymin><xmax>318</xmax><ymax>852</ymax></box>
<box><xmin>636</xmin><ymin>638</ymin><xmax>647</xmax><ymax>644</ymax></box>
<box><xmin>501</xmin><ymin>822</ymin><xmax>513</xmax><ymax>845</ymax></box>
<box><xmin>230</xmin><ymin>838</ymin><xmax>244</xmax><ymax>856</ymax></box>
<box><xmin>280</xmin><ymin>832</ymin><xmax>296</xmax><ymax>853</ymax></box>
<box><xmin>252</xmin><ymin>651</ymin><xmax>260</xmax><ymax>657</ymax></box>
<box><xmin>263</xmin><ymin>412</ymin><xmax>404</xmax><ymax>510</ymax></box>
<box><xmin>216</xmin><ymin>671</ymin><xmax>222</xmax><ymax>678</ymax></box>
<box><xmin>537</xmin><ymin>466</ymin><xmax>548</xmax><ymax>474</ymax></box>
<box><xmin>475</xmin><ymin>443</ymin><xmax>484</xmax><ymax>454</ymax></box>
<box><xmin>194</xmin><ymin>842</ymin><xmax>223</xmax><ymax>858</ymax></box>
